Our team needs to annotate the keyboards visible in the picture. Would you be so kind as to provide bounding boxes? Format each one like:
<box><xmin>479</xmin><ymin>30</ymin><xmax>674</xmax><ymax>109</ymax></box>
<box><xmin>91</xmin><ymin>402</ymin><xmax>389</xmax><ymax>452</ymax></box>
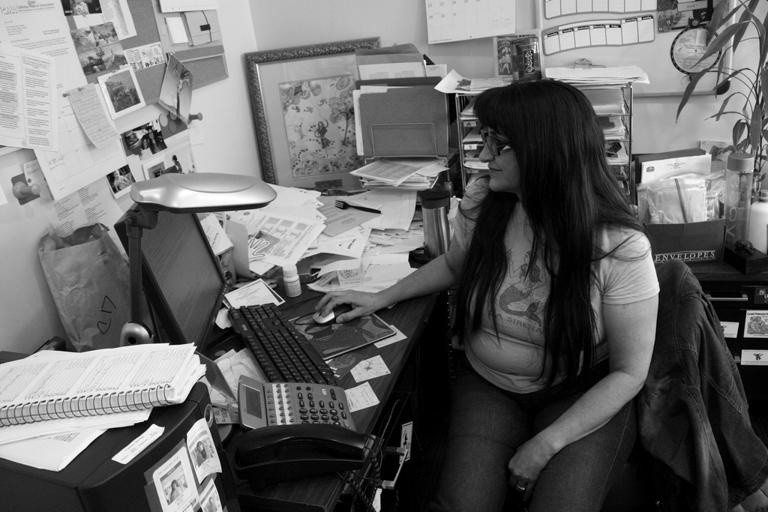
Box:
<box><xmin>227</xmin><ymin>302</ymin><xmax>337</xmax><ymax>385</ymax></box>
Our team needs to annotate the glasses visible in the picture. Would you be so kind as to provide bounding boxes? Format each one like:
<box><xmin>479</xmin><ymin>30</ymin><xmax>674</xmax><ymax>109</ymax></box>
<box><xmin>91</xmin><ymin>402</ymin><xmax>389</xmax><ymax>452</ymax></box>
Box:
<box><xmin>480</xmin><ymin>130</ymin><xmax>513</xmax><ymax>156</ymax></box>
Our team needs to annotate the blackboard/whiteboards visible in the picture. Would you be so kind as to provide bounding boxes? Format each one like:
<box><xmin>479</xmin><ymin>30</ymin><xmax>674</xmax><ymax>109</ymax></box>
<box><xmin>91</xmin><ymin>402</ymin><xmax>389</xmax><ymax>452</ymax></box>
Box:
<box><xmin>534</xmin><ymin>0</ymin><xmax>737</xmax><ymax>99</ymax></box>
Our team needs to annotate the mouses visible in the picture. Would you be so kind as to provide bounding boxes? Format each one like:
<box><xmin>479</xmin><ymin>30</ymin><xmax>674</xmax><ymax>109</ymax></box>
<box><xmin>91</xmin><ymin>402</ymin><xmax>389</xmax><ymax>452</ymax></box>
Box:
<box><xmin>312</xmin><ymin>303</ymin><xmax>353</xmax><ymax>324</ymax></box>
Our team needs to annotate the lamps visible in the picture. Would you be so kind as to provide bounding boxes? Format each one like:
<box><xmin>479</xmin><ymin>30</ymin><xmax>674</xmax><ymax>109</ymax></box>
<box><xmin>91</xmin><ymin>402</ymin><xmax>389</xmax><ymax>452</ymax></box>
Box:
<box><xmin>117</xmin><ymin>171</ymin><xmax>275</xmax><ymax>347</ymax></box>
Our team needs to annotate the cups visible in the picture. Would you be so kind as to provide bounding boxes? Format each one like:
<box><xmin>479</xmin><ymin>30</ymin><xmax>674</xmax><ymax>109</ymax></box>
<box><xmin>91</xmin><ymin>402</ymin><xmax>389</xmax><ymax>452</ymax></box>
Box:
<box><xmin>727</xmin><ymin>150</ymin><xmax>755</xmax><ymax>256</ymax></box>
<box><xmin>420</xmin><ymin>189</ymin><xmax>452</xmax><ymax>262</ymax></box>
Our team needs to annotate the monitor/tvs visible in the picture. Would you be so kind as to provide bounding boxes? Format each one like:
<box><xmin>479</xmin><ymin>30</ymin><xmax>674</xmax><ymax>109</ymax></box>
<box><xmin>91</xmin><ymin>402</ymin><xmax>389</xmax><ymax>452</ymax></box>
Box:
<box><xmin>115</xmin><ymin>166</ymin><xmax>228</xmax><ymax>359</ymax></box>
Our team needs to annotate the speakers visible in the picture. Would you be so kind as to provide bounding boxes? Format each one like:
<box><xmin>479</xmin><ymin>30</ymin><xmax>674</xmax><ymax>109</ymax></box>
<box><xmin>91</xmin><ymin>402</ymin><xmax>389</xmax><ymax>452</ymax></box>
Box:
<box><xmin>217</xmin><ymin>249</ymin><xmax>236</xmax><ymax>286</ymax></box>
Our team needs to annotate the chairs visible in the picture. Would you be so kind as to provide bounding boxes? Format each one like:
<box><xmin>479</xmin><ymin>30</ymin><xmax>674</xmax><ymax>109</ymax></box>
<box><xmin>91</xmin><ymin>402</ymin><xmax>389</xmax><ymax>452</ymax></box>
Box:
<box><xmin>602</xmin><ymin>259</ymin><xmax>733</xmax><ymax>508</ymax></box>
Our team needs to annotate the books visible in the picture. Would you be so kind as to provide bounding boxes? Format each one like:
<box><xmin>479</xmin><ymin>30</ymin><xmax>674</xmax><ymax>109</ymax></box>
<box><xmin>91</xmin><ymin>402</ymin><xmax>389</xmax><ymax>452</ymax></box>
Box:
<box><xmin>0</xmin><ymin>340</ymin><xmax>208</xmax><ymax>427</ymax></box>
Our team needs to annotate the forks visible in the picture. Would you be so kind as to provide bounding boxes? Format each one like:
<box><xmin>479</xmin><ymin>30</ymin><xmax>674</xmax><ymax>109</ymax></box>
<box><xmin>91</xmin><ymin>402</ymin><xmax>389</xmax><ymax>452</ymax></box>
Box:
<box><xmin>335</xmin><ymin>198</ymin><xmax>381</xmax><ymax>215</ymax></box>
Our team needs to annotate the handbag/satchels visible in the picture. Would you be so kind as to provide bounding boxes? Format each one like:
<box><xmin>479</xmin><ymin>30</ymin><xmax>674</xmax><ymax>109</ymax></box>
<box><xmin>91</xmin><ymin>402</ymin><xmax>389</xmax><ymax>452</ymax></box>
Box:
<box><xmin>37</xmin><ymin>224</ymin><xmax>132</xmax><ymax>350</ymax></box>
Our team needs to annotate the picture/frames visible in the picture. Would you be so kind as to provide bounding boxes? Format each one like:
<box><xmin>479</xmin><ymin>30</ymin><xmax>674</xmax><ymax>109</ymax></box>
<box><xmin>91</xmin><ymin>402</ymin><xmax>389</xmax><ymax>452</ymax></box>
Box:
<box><xmin>243</xmin><ymin>34</ymin><xmax>381</xmax><ymax>195</ymax></box>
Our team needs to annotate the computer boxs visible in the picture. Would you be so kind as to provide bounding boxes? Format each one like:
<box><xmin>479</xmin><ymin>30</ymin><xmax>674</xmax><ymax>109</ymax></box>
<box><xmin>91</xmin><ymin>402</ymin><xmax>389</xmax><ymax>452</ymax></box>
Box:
<box><xmin>0</xmin><ymin>350</ymin><xmax>231</xmax><ymax>512</ymax></box>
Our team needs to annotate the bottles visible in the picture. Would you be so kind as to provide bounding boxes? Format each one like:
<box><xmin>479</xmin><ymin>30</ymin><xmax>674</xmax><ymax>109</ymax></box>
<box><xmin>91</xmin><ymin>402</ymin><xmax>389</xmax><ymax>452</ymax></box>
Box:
<box><xmin>750</xmin><ymin>189</ymin><xmax>768</xmax><ymax>254</ymax></box>
<box><xmin>281</xmin><ymin>263</ymin><xmax>302</xmax><ymax>296</ymax></box>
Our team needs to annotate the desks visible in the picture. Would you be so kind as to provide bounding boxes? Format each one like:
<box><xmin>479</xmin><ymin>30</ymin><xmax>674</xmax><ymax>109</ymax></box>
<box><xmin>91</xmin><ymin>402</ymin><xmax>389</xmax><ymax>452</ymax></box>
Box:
<box><xmin>689</xmin><ymin>261</ymin><xmax>768</xmax><ymax>447</ymax></box>
<box><xmin>205</xmin><ymin>281</ymin><xmax>439</xmax><ymax>510</ymax></box>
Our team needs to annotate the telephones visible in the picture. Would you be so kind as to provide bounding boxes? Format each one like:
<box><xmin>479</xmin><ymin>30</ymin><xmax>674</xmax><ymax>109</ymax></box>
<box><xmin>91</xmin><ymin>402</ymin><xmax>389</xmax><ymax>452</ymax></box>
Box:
<box><xmin>231</xmin><ymin>375</ymin><xmax>366</xmax><ymax>493</ymax></box>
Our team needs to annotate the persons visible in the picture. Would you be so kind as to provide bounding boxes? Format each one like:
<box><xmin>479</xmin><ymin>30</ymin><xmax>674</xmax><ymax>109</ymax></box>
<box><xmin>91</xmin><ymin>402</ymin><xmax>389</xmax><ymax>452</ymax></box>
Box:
<box><xmin>315</xmin><ymin>79</ymin><xmax>661</xmax><ymax>511</ymax></box>
<box><xmin>145</xmin><ymin>126</ymin><xmax>165</xmax><ymax>153</ymax></box>
<box><xmin>112</xmin><ymin>169</ymin><xmax>132</xmax><ymax>190</ymax></box>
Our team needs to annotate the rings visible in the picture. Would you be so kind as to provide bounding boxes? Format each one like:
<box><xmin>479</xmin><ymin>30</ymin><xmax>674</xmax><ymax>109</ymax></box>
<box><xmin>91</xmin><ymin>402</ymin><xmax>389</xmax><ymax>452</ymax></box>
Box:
<box><xmin>516</xmin><ymin>484</ymin><xmax>525</xmax><ymax>491</ymax></box>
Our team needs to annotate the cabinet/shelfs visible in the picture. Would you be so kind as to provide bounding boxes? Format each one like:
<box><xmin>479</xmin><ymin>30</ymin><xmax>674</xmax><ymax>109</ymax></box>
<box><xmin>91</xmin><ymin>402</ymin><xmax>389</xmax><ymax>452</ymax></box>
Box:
<box><xmin>454</xmin><ymin>80</ymin><xmax>635</xmax><ymax>210</ymax></box>
